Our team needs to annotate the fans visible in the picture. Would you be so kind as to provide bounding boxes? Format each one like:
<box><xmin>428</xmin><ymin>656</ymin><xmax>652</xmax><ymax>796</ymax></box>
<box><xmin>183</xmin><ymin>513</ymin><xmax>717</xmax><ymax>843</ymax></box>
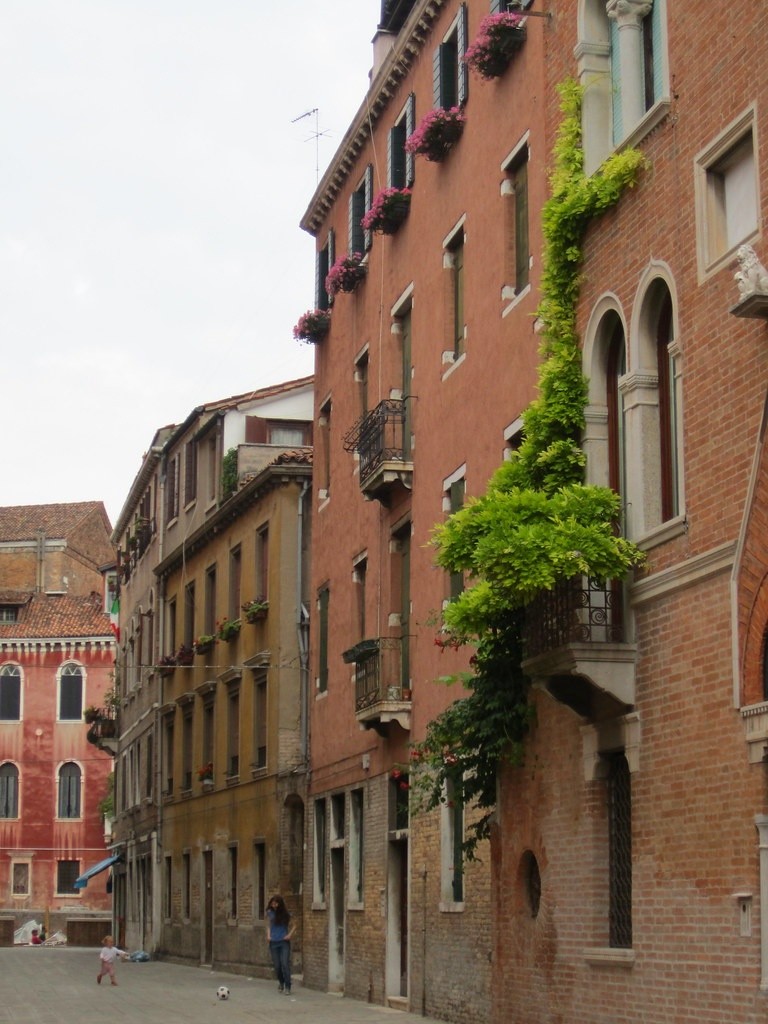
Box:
<box><xmin>214</xmin><ymin>617</ymin><xmax>241</xmax><ymax>641</ymax></box>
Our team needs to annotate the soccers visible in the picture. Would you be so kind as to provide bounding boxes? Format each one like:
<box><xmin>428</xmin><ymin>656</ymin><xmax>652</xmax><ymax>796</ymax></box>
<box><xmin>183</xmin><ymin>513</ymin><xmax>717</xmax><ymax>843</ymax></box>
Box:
<box><xmin>216</xmin><ymin>986</ymin><xmax>231</xmax><ymax>1000</ymax></box>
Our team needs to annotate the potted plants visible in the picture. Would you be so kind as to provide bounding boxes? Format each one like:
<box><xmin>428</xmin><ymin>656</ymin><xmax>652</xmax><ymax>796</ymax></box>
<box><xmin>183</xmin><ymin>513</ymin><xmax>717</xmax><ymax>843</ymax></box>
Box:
<box><xmin>107</xmin><ymin>578</ymin><xmax>115</xmax><ymax>592</ymax></box>
<box><xmin>101</xmin><ymin>718</ymin><xmax>115</xmax><ymax>738</ymax></box>
<box><xmin>87</xmin><ymin>705</ymin><xmax>98</xmax><ymax>720</ymax></box>
<box><xmin>127</xmin><ymin>537</ymin><xmax>138</xmax><ymax>551</ymax></box>
<box><xmin>120</xmin><ymin>551</ymin><xmax>131</xmax><ymax>563</ymax></box>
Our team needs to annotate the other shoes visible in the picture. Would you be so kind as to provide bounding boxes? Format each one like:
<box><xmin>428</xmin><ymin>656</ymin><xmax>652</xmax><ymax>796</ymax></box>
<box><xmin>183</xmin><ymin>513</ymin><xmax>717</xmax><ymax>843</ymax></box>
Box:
<box><xmin>285</xmin><ymin>986</ymin><xmax>291</xmax><ymax>995</ymax></box>
<box><xmin>97</xmin><ymin>975</ymin><xmax>102</xmax><ymax>983</ymax></box>
<box><xmin>111</xmin><ymin>981</ymin><xmax>119</xmax><ymax>986</ymax></box>
<box><xmin>278</xmin><ymin>984</ymin><xmax>283</xmax><ymax>993</ymax></box>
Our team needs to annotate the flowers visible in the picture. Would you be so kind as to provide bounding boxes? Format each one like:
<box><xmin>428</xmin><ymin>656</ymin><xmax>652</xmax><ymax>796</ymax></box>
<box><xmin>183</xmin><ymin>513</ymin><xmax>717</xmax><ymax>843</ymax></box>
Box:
<box><xmin>403</xmin><ymin>106</ymin><xmax>468</xmax><ymax>162</ymax></box>
<box><xmin>460</xmin><ymin>10</ymin><xmax>528</xmax><ymax>81</ymax></box>
<box><xmin>193</xmin><ymin>633</ymin><xmax>220</xmax><ymax>649</ymax></box>
<box><xmin>324</xmin><ymin>251</ymin><xmax>369</xmax><ymax>295</ymax></box>
<box><xmin>153</xmin><ymin>655</ymin><xmax>177</xmax><ymax>673</ymax></box>
<box><xmin>292</xmin><ymin>308</ymin><xmax>332</xmax><ymax>347</ymax></box>
<box><xmin>174</xmin><ymin>642</ymin><xmax>194</xmax><ymax>666</ymax></box>
<box><xmin>360</xmin><ymin>186</ymin><xmax>413</xmax><ymax>235</ymax></box>
<box><xmin>194</xmin><ymin>762</ymin><xmax>213</xmax><ymax>781</ymax></box>
<box><xmin>242</xmin><ymin>598</ymin><xmax>269</xmax><ymax>618</ymax></box>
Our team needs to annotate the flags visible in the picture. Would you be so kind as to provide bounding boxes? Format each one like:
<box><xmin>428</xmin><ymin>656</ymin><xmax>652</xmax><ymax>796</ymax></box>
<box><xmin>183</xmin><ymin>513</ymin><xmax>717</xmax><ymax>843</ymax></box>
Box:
<box><xmin>110</xmin><ymin>596</ymin><xmax>120</xmax><ymax>643</ymax></box>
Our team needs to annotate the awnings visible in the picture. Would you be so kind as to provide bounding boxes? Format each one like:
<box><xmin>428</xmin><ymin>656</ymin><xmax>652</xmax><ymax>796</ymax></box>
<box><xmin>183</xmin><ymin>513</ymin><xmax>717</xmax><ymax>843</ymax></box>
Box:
<box><xmin>74</xmin><ymin>855</ymin><xmax>121</xmax><ymax>888</ymax></box>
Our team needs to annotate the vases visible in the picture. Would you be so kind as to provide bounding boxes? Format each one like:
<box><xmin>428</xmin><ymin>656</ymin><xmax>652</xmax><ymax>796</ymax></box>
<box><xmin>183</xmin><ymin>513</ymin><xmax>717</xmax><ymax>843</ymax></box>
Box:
<box><xmin>201</xmin><ymin>778</ymin><xmax>212</xmax><ymax>785</ymax></box>
<box><xmin>160</xmin><ymin>668</ymin><xmax>174</xmax><ymax>677</ymax></box>
<box><xmin>427</xmin><ymin>121</ymin><xmax>463</xmax><ymax>160</ymax></box>
<box><xmin>247</xmin><ymin>611</ymin><xmax>267</xmax><ymax>623</ymax></box>
<box><xmin>381</xmin><ymin>202</ymin><xmax>407</xmax><ymax>234</ymax></box>
<box><xmin>178</xmin><ymin>655</ymin><xmax>193</xmax><ymax>665</ymax></box>
<box><xmin>486</xmin><ymin>29</ymin><xmax>524</xmax><ymax>75</ymax></box>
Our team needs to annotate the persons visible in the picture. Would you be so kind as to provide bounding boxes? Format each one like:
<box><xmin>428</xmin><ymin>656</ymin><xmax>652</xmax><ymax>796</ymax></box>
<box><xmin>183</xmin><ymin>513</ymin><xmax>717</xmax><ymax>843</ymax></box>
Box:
<box><xmin>31</xmin><ymin>929</ymin><xmax>43</xmax><ymax>944</ymax></box>
<box><xmin>39</xmin><ymin>926</ymin><xmax>47</xmax><ymax>942</ymax></box>
<box><xmin>97</xmin><ymin>935</ymin><xmax>130</xmax><ymax>986</ymax></box>
<box><xmin>265</xmin><ymin>895</ymin><xmax>297</xmax><ymax>995</ymax></box>
